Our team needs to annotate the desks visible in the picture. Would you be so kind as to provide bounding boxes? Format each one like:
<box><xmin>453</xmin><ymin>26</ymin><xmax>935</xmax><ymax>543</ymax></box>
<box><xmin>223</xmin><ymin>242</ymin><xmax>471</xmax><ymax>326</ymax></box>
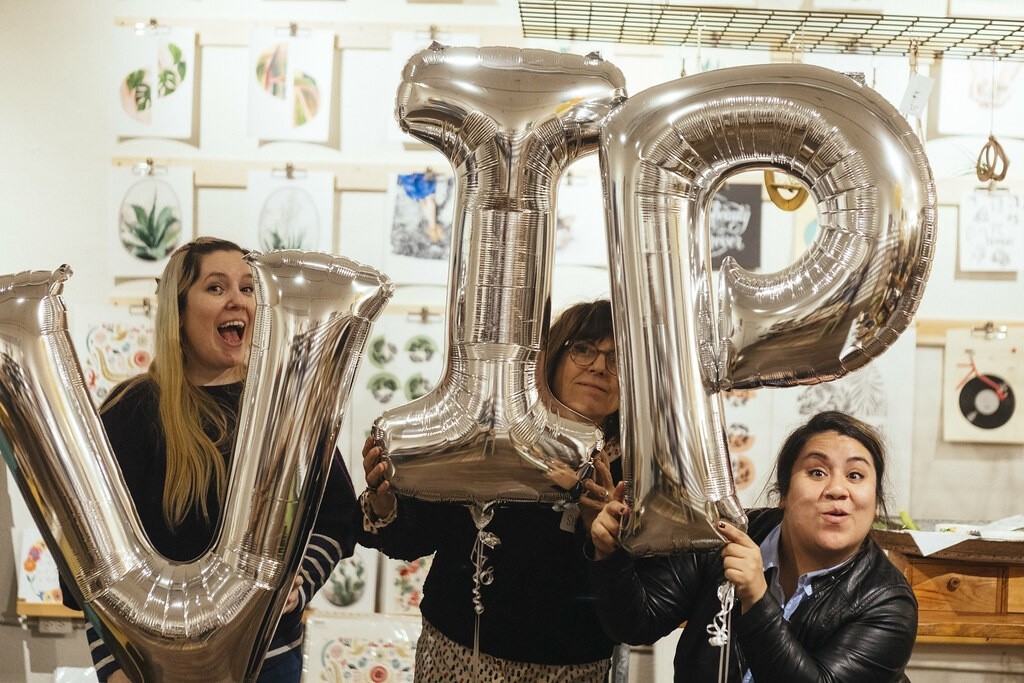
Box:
<box><xmin>868</xmin><ymin>531</ymin><xmax>1024</xmax><ymax>647</ymax></box>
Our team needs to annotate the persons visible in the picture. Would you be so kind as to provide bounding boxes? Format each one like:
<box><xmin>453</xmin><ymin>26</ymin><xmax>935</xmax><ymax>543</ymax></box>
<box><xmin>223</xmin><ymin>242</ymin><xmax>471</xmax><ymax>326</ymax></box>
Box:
<box><xmin>359</xmin><ymin>299</ymin><xmax>622</xmax><ymax>682</ymax></box>
<box><xmin>591</xmin><ymin>410</ymin><xmax>917</xmax><ymax>682</ymax></box>
<box><xmin>57</xmin><ymin>236</ymin><xmax>359</xmax><ymax>683</ymax></box>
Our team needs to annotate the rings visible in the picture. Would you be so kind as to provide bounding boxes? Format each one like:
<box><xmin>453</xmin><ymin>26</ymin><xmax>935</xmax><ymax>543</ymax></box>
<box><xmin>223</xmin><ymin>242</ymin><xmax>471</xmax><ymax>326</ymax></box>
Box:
<box><xmin>605</xmin><ymin>491</ymin><xmax>608</xmax><ymax>501</ymax></box>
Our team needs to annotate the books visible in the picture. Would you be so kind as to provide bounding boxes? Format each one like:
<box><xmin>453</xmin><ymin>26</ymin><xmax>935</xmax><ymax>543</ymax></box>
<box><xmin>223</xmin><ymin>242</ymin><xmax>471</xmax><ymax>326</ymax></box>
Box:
<box><xmin>935</xmin><ymin>523</ymin><xmax>1024</xmax><ymax>542</ymax></box>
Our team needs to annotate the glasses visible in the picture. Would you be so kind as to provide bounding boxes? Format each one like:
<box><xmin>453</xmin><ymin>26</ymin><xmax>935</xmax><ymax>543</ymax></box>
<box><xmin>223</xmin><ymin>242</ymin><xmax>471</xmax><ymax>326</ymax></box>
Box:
<box><xmin>564</xmin><ymin>339</ymin><xmax>617</xmax><ymax>376</ymax></box>
